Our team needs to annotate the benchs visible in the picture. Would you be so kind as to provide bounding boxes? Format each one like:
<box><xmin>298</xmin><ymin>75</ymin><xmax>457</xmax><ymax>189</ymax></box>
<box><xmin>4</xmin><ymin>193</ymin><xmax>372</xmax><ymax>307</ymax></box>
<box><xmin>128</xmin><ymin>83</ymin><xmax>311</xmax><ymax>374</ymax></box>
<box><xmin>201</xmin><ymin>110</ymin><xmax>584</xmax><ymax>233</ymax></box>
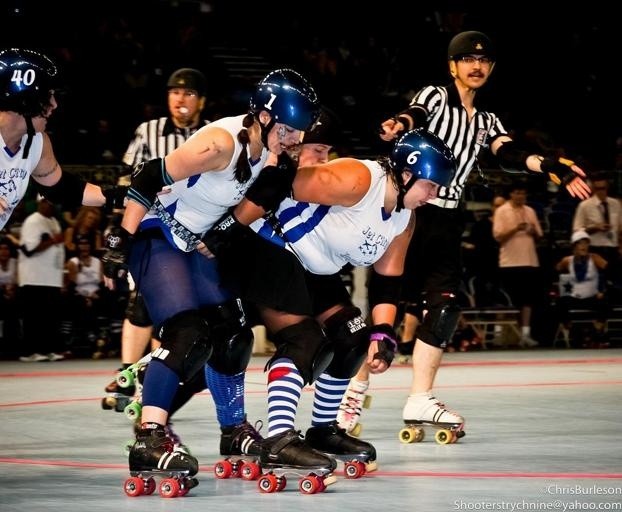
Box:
<box><xmin>545</xmin><ymin>298</ymin><xmax>621</xmax><ymax>349</ymax></box>
<box><xmin>455</xmin><ymin>307</ymin><xmax>520</xmax><ymax>349</ymax></box>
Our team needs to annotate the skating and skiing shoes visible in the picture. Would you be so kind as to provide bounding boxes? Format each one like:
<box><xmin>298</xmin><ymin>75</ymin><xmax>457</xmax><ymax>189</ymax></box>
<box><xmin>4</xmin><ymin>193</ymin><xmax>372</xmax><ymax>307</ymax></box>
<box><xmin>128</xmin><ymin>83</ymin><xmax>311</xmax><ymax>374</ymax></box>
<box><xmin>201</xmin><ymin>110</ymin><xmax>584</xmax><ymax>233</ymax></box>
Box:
<box><xmin>336</xmin><ymin>378</ymin><xmax>372</xmax><ymax>437</ymax></box>
<box><xmin>124</xmin><ymin>420</ymin><xmax>199</xmax><ymax>498</ymax></box>
<box><xmin>214</xmin><ymin>419</ymin><xmax>377</xmax><ymax>495</ymax></box>
<box><xmin>398</xmin><ymin>394</ymin><xmax>465</xmax><ymax>444</ymax></box>
<box><xmin>102</xmin><ymin>351</ymin><xmax>152</xmax><ymax>421</ymax></box>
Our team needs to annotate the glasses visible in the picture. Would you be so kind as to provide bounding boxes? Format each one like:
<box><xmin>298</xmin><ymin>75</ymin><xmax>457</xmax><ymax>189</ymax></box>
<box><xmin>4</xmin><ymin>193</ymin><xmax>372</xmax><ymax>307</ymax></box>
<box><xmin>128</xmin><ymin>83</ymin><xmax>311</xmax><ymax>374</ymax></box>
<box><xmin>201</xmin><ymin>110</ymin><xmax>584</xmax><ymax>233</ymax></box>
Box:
<box><xmin>463</xmin><ymin>55</ymin><xmax>489</xmax><ymax>64</ymax></box>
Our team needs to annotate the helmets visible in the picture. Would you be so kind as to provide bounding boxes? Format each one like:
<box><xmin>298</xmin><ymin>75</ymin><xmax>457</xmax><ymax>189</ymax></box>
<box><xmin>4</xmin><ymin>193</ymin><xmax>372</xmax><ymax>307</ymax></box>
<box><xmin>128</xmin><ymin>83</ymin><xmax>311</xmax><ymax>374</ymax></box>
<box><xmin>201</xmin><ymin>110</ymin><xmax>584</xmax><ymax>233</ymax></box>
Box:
<box><xmin>168</xmin><ymin>68</ymin><xmax>206</xmax><ymax>88</ymax></box>
<box><xmin>250</xmin><ymin>68</ymin><xmax>323</xmax><ymax>132</ymax></box>
<box><xmin>0</xmin><ymin>48</ymin><xmax>59</xmax><ymax>118</ymax></box>
<box><xmin>389</xmin><ymin>127</ymin><xmax>457</xmax><ymax>187</ymax></box>
<box><xmin>448</xmin><ymin>31</ymin><xmax>494</xmax><ymax>59</ymax></box>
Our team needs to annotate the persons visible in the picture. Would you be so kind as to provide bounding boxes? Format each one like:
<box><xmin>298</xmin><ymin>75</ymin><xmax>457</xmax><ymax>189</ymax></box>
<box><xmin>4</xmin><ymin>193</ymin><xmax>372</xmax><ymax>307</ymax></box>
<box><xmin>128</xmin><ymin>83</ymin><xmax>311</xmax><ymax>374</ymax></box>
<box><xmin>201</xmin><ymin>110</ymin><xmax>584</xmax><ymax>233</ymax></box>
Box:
<box><xmin>128</xmin><ymin>111</ymin><xmax>339</xmax><ymax>445</ymax></box>
<box><xmin>333</xmin><ymin>28</ymin><xmax>595</xmax><ymax>426</ymax></box>
<box><xmin>194</xmin><ymin>130</ymin><xmax>458</xmax><ymax>468</ymax></box>
<box><xmin>106</xmin><ymin>67</ymin><xmax>217</xmax><ymax>396</ymax></box>
<box><xmin>97</xmin><ymin>64</ymin><xmax>324</xmax><ymax>475</ymax></box>
<box><xmin>1</xmin><ymin>150</ymin><xmax>621</xmax><ymax>366</ymax></box>
<box><xmin>1</xmin><ymin>46</ymin><xmax>176</xmax><ymax>244</ymax></box>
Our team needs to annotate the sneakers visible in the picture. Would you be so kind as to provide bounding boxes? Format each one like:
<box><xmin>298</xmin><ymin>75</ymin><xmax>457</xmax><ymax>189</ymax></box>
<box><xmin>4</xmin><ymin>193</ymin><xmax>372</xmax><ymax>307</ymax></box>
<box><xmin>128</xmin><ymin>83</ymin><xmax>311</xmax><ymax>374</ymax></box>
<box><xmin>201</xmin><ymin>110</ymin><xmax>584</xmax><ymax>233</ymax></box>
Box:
<box><xmin>19</xmin><ymin>353</ymin><xmax>65</xmax><ymax>362</ymax></box>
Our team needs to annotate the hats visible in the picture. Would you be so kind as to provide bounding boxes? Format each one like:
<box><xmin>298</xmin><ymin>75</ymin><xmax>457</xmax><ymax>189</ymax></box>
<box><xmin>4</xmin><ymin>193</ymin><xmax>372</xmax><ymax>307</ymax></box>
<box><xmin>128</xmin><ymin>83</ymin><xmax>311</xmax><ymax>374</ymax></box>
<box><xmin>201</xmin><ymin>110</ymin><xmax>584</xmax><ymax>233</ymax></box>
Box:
<box><xmin>571</xmin><ymin>231</ymin><xmax>591</xmax><ymax>244</ymax></box>
